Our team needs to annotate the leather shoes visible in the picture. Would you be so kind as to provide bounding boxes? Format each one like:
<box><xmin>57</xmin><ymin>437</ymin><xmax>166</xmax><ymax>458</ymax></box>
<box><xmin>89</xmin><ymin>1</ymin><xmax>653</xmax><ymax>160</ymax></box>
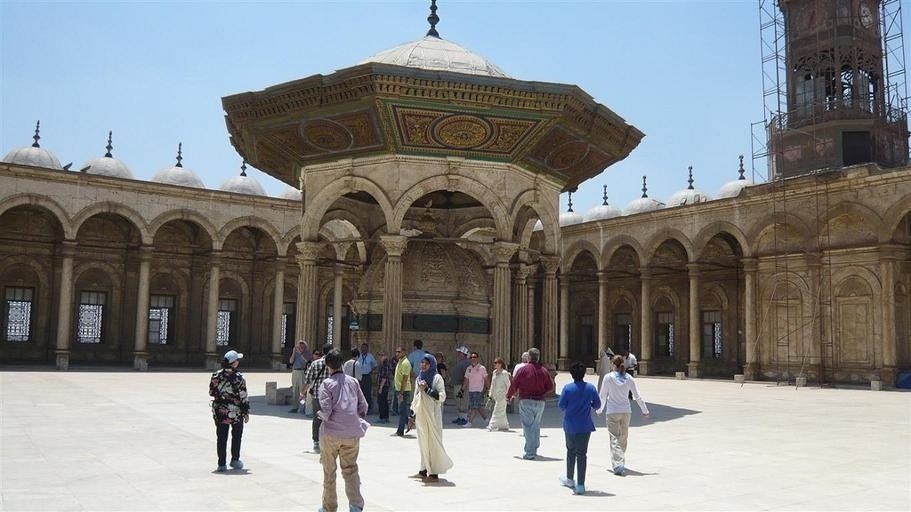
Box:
<box><xmin>390</xmin><ymin>421</ymin><xmax>415</xmax><ymax>436</ymax></box>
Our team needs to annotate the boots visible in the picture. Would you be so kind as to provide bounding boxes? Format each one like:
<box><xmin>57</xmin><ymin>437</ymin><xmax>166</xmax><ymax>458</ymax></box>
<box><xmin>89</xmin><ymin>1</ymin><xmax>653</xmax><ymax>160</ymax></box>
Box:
<box><xmin>417</xmin><ymin>470</ymin><xmax>438</xmax><ymax>482</ymax></box>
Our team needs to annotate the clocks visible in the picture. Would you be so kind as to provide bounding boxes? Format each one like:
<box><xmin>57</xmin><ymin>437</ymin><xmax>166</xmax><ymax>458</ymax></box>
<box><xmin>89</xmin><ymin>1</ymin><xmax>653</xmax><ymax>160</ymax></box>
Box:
<box><xmin>859</xmin><ymin>2</ymin><xmax>874</xmax><ymax>29</ymax></box>
<box><xmin>797</xmin><ymin>2</ymin><xmax>828</xmax><ymax>34</ymax></box>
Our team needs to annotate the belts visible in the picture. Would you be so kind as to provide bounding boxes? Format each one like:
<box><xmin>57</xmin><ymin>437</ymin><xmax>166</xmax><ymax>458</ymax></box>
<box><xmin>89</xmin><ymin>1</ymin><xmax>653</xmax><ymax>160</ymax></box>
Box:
<box><xmin>294</xmin><ymin>368</ymin><xmax>305</xmax><ymax>370</ymax></box>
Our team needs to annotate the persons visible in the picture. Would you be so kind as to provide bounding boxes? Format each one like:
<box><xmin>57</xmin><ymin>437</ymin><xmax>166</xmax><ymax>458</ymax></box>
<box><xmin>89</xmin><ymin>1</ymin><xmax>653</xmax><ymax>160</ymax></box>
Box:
<box><xmin>597</xmin><ymin>347</ymin><xmax>638</xmax><ymax>400</ymax></box>
<box><xmin>289</xmin><ymin>339</ymin><xmax>553</xmax><ymax>458</ymax></box>
<box><xmin>407</xmin><ymin>353</ymin><xmax>455</xmax><ymax>482</ymax></box>
<box><xmin>596</xmin><ymin>354</ymin><xmax>649</xmax><ymax>475</ymax></box>
<box><xmin>558</xmin><ymin>360</ymin><xmax>601</xmax><ymax>494</ymax></box>
<box><xmin>316</xmin><ymin>347</ymin><xmax>371</xmax><ymax>512</ymax></box>
<box><xmin>208</xmin><ymin>351</ymin><xmax>250</xmax><ymax>472</ymax></box>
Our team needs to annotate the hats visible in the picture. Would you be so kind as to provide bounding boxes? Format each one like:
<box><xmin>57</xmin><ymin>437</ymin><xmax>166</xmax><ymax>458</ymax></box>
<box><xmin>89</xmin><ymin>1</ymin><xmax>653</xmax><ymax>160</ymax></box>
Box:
<box><xmin>456</xmin><ymin>345</ymin><xmax>469</xmax><ymax>355</ymax></box>
<box><xmin>224</xmin><ymin>349</ymin><xmax>243</xmax><ymax>364</ymax></box>
<box><xmin>297</xmin><ymin>340</ymin><xmax>308</xmax><ymax>350</ymax></box>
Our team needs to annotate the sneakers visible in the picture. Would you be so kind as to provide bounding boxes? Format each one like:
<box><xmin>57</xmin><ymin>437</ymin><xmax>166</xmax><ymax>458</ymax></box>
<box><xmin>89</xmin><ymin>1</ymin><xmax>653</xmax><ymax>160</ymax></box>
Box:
<box><xmin>523</xmin><ymin>453</ymin><xmax>535</xmax><ymax>460</ymax></box>
<box><xmin>218</xmin><ymin>458</ymin><xmax>241</xmax><ymax>471</ymax></box>
<box><xmin>608</xmin><ymin>465</ymin><xmax>625</xmax><ymax>475</ymax></box>
<box><xmin>453</xmin><ymin>416</ymin><xmax>510</xmax><ymax>431</ymax></box>
<box><xmin>314</xmin><ymin>441</ymin><xmax>319</xmax><ymax>447</ymax></box>
<box><xmin>559</xmin><ymin>476</ymin><xmax>585</xmax><ymax>494</ymax></box>
<box><xmin>319</xmin><ymin>507</ymin><xmax>362</xmax><ymax>512</ymax></box>
<box><xmin>373</xmin><ymin>418</ymin><xmax>389</xmax><ymax>424</ymax></box>
<box><xmin>288</xmin><ymin>408</ymin><xmax>313</xmax><ymax>418</ymax></box>
